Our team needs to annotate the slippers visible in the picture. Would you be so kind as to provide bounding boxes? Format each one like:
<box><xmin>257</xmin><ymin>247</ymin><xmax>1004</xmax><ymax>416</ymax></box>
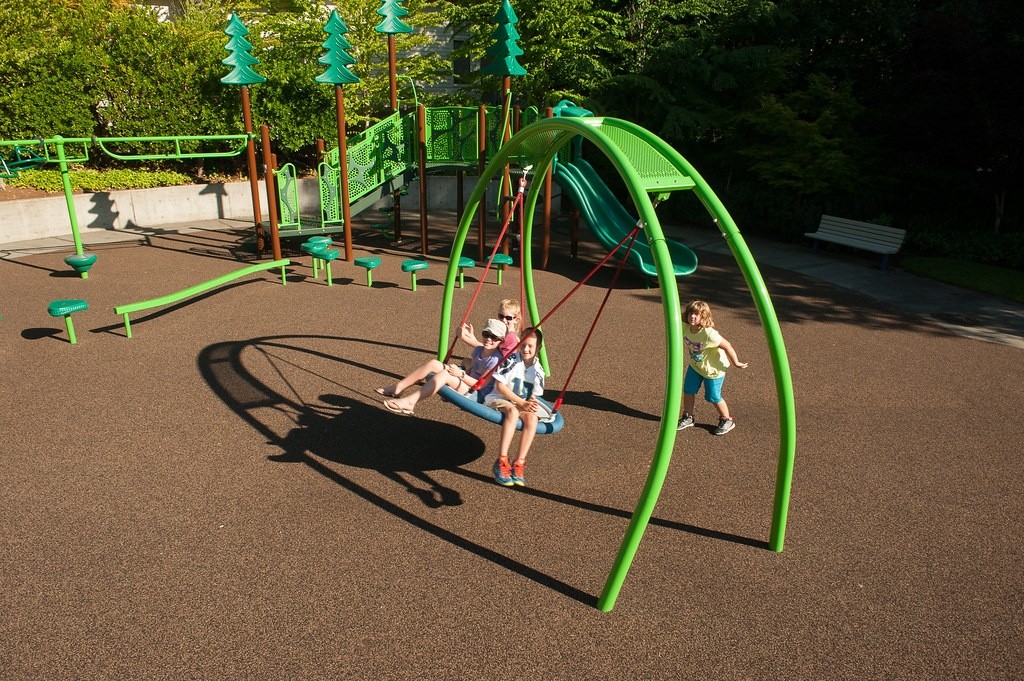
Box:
<box><xmin>383</xmin><ymin>399</ymin><xmax>414</xmax><ymax>415</ymax></box>
<box><xmin>373</xmin><ymin>386</ymin><xmax>401</xmax><ymax>398</ymax></box>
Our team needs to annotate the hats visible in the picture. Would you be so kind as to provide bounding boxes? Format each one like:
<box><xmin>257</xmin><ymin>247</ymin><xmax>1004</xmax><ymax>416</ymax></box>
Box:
<box><xmin>482</xmin><ymin>319</ymin><xmax>507</xmax><ymax>337</ymax></box>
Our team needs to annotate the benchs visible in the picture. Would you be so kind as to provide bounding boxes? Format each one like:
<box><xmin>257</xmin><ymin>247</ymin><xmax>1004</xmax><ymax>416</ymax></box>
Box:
<box><xmin>804</xmin><ymin>214</ymin><xmax>906</xmax><ymax>269</ymax></box>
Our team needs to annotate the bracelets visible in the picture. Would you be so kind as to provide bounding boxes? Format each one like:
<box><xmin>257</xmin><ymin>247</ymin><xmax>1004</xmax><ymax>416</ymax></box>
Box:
<box><xmin>459</xmin><ymin>370</ymin><xmax>466</xmax><ymax>380</ymax></box>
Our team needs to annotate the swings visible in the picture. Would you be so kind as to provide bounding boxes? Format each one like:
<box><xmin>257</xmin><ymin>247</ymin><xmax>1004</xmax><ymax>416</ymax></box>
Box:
<box><xmin>425</xmin><ymin>176</ymin><xmax>645</xmax><ymax>436</ymax></box>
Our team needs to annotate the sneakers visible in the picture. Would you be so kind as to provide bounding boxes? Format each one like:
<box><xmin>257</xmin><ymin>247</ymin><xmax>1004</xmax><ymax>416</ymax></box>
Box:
<box><xmin>677</xmin><ymin>411</ymin><xmax>695</xmax><ymax>431</ymax></box>
<box><xmin>493</xmin><ymin>457</ymin><xmax>514</xmax><ymax>486</ymax></box>
<box><xmin>511</xmin><ymin>460</ymin><xmax>527</xmax><ymax>486</ymax></box>
<box><xmin>714</xmin><ymin>416</ymin><xmax>736</xmax><ymax>436</ymax></box>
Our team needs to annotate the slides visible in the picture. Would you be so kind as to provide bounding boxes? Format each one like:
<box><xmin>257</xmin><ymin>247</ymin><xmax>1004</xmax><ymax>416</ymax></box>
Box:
<box><xmin>553</xmin><ymin>155</ymin><xmax>700</xmax><ymax>279</ymax></box>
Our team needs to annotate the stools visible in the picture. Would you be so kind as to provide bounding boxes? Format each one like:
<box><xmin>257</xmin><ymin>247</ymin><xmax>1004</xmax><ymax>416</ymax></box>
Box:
<box><xmin>487</xmin><ymin>253</ymin><xmax>513</xmax><ymax>285</ymax></box>
<box><xmin>458</xmin><ymin>257</ymin><xmax>475</xmax><ymax>288</ymax></box>
<box><xmin>302</xmin><ymin>243</ymin><xmax>327</xmax><ymax>278</ymax></box>
<box><xmin>46</xmin><ymin>298</ymin><xmax>88</xmax><ymax>343</ymax></box>
<box><xmin>307</xmin><ymin>236</ymin><xmax>333</xmax><ymax>270</ymax></box>
<box><xmin>313</xmin><ymin>249</ymin><xmax>341</xmax><ymax>285</ymax></box>
<box><xmin>401</xmin><ymin>259</ymin><xmax>429</xmax><ymax>291</ymax></box>
<box><xmin>354</xmin><ymin>257</ymin><xmax>381</xmax><ymax>286</ymax></box>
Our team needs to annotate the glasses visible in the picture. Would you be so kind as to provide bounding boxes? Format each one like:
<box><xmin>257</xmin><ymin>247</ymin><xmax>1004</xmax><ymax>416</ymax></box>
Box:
<box><xmin>497</xmin><ymin>313</ymin><xmax>517</xmax><ymax>321</ymax></box>
<box><xmin>482</xmin><ymin>331</ymin><xmax>502</xmax><ymax>342</ymax></box>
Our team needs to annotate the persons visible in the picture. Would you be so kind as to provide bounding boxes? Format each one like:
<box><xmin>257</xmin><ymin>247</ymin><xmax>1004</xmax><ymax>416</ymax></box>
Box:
<box><xmin>372</xmin><ymin>318</ymin><xmax>506</xmax><ymax>415</ymax></box>
<box><xmin>676</xmin><ymin>300</ymin><xmax>748</xmax><ymax>435</ymax></box>
<box><xmin>455</xmin><ymin>298</ymin><xmax>521</xmax><ymax>357</ymax></box>
<box><xmin>481</xmin><ymin>327</ymin><xmax>544</xmax><ymax>487</ymax></box>
